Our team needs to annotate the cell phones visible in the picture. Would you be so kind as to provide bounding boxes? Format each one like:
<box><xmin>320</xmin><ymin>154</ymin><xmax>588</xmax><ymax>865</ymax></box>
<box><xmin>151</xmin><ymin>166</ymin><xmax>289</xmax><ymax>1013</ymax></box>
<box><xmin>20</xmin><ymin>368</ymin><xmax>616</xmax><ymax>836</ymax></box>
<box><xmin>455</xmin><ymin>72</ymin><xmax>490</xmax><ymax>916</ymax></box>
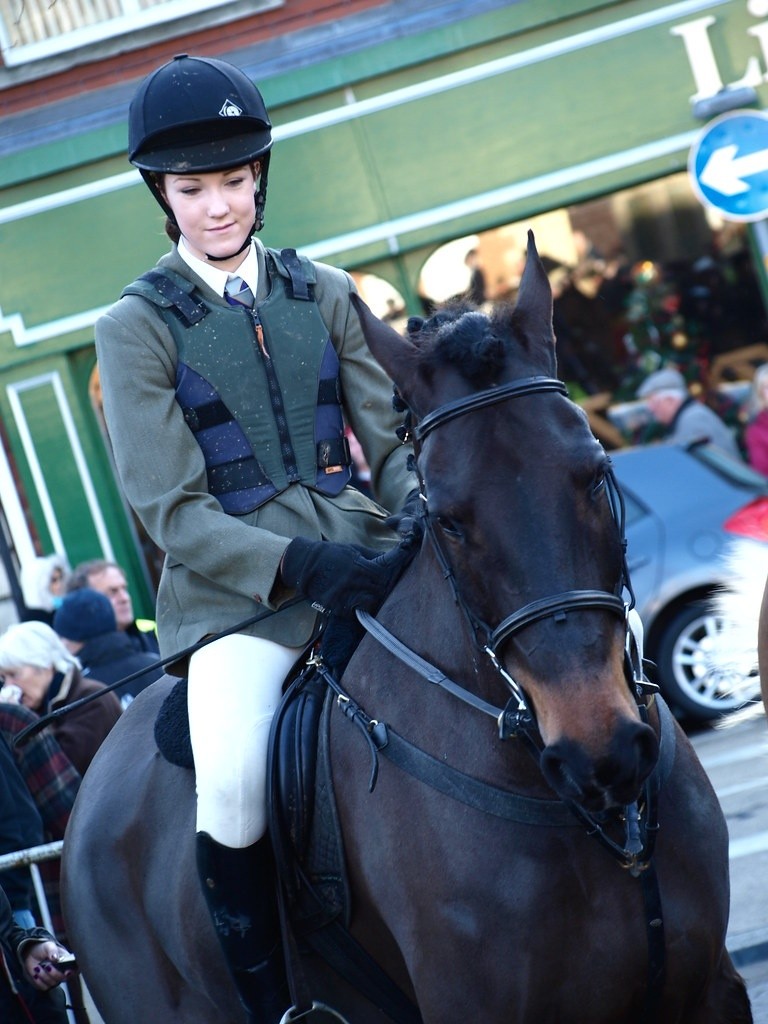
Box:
<box><xmin>39</xmin><ymin>953</ymin><xmax>78</xmax><ymax>971</ymax></box>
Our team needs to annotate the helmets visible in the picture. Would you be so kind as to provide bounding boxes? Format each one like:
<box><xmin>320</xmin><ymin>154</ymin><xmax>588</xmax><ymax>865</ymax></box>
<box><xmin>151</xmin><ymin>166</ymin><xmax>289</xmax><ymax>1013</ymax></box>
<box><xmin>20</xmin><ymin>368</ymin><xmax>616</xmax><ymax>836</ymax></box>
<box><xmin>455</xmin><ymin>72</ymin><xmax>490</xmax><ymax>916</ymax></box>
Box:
<box><xmin>130</xmin><ymin>53</ymin><xmax>274</xmax><ymax>174</ymax></box>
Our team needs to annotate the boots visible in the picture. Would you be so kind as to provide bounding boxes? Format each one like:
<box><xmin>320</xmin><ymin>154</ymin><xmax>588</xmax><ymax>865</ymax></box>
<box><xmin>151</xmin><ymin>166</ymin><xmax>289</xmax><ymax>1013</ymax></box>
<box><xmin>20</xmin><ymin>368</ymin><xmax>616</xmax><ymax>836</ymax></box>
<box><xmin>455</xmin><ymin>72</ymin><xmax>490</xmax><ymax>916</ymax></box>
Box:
<box><xmin>195</xmin><ymin>829</ymin><xmax>284</xmax><ymax>1024</ymax></box>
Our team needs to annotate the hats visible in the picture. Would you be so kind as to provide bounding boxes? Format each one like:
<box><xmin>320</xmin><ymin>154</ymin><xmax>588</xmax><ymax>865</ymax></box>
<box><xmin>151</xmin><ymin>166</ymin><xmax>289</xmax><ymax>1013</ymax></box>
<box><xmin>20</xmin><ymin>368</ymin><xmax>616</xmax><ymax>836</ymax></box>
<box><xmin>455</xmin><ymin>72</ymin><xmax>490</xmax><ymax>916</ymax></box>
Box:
<box><xmin>53</xmin><ymin>589</ymin><xmax>116</xmax><ymax>643</ymax></box>
<box><xmin>635</xmin><ymin>370</ymin><xmax>685</xmax><ymax>398</ymax></box>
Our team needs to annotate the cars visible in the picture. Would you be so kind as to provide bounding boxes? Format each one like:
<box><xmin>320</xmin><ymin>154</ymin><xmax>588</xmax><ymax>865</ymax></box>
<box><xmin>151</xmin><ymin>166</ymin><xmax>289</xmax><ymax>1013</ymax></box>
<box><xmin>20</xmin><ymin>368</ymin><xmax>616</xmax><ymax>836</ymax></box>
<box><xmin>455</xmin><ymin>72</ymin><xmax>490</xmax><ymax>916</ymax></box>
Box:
<box><xmin>603</xmin><ymin>436</ymin><xmax>767</xmax><ymax>724</ymax></box>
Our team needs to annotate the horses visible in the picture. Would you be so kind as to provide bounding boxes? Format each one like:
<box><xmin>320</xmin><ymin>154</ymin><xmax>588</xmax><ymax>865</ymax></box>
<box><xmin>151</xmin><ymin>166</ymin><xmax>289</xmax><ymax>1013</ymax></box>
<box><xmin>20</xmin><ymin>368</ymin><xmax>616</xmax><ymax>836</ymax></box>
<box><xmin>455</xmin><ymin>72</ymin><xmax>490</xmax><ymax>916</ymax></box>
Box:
<box><xmin>56</xmin><ymin>227</ymin><xmax>757</xmax><ymax>1024</ymax></box>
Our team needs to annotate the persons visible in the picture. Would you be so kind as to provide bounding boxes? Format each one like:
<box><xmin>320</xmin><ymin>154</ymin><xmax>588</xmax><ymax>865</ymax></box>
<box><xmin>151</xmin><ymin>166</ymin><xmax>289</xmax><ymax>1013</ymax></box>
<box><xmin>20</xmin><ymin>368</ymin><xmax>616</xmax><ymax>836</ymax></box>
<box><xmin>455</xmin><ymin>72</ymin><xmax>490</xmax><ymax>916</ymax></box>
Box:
<box><xmin>0</xmin><ymin>553</ymin><xmax>164</xmax><ymax>1024</ymax></box>
<box><xmin>744</xmin><ymin>363</ymin><xmax>768</xmax><ymax>474</ymax></box>
<box><xmin>637</xmin><ymin>369</ymin><xmax>746</xmax><ymax>468</ymax></box>
<box><xmin>94</xmin><ymin>52</ymin><xmax>420</xmax><ymax>1024</ymax></box>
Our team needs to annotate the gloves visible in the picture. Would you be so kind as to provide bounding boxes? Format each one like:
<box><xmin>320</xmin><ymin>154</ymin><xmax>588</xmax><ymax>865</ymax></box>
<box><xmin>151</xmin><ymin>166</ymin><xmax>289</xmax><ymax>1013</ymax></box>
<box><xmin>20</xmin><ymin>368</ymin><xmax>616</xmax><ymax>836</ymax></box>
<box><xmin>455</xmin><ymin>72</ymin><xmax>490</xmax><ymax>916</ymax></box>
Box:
<box><xmin>385</xmin><ymin>488</ymin><xmax>430</xmax><ymax>546</ymax></box>
<box><xmin>281</xmin><ymin>538</ymin><xmax>385</xmax><ymax>617</ymax></box>
